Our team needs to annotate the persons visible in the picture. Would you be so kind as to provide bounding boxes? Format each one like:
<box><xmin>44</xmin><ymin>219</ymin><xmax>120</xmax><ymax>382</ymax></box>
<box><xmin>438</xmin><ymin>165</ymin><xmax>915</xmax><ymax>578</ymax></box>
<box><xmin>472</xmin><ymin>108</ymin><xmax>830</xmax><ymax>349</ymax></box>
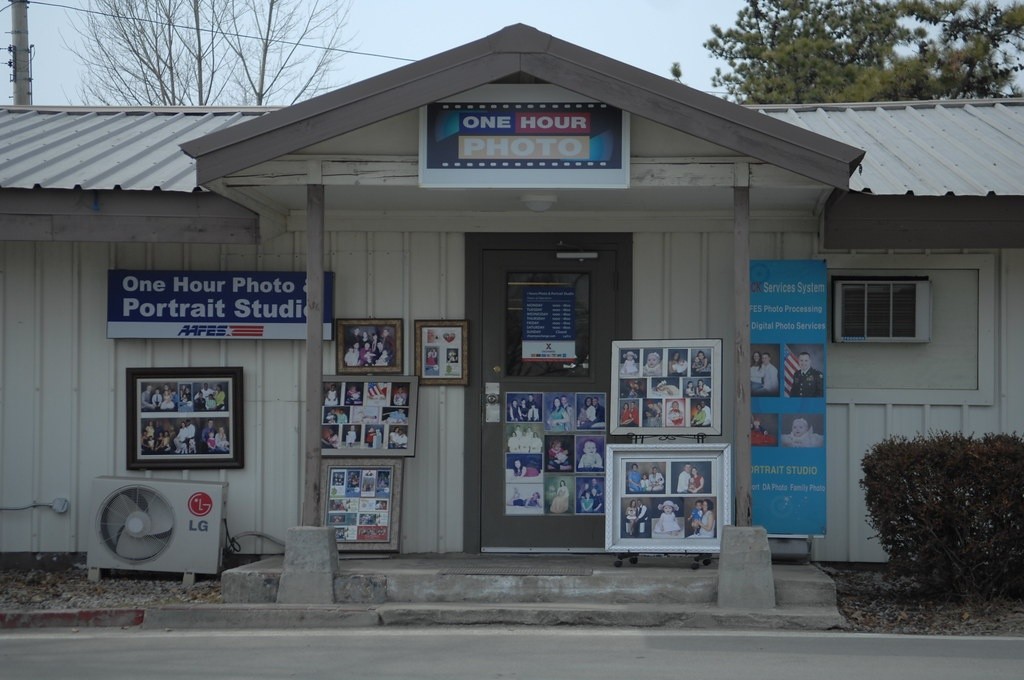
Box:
<box><xmin>789</xmin><ymin>352</ymin><xmax>824</xmax><ymax>398</ymax></box>
<box><xmin>425</xmin><ymin>343</ymin><xmax>460</xmax><ymax>362</ymax></box>
<box><xmin>620</xmin><ymin>349</ymin><xmax>712</xmax><ymax>426</ymax></box>
<box><xmin>622</xmin><ymin>460</ymin><xmax>718</xmax><ymax>539</ymax></box>
<box><xmin>750</xmin><ymin>351</ymin><xmax>780</xmax><ymax>394</ymax></box>
<box><xmin>141</xmin><ymin>418</ymin><xmax>230</xmax><ymax>453</ymax></box>
<box><xmin>781</xmin><ymin>419</ymin><xmax>824</xmax><ymax>447</ymax></box>
<box><xmin>323</xmin><ymin>383</ymin><xmax>409</xmax><ymax>448</ymax></box>
<box><xmin>344</xmin><ymin>326</ymin><xmax>396</xmax><ymax>367</ymax></box>
<box><xmin>506</xmin><ymin>394</ymin><xmax>605</xmax><ymax>516</ymax></box>
<box><xmin>142</xmin><ymin>383</ymin><xmax>229</xmax><ymax>411</ymax></box>
<box><xmin>330</xmin><ymin>469</ymin><xmax>391</xmax><ymax>539</ymax></box>
<box><xmin>751</xmin><ymin>418</ymin><xmax>770</xmax><ymax>435</ymax></box>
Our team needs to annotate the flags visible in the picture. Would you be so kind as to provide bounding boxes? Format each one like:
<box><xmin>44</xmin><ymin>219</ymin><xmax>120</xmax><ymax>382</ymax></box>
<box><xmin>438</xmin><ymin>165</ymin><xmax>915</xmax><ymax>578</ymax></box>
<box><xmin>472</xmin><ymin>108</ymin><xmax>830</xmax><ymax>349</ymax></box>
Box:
<box><xmin>784</xmin><ymin>345</ymin><xmax>801</xmax><ymax>396</ymax></box>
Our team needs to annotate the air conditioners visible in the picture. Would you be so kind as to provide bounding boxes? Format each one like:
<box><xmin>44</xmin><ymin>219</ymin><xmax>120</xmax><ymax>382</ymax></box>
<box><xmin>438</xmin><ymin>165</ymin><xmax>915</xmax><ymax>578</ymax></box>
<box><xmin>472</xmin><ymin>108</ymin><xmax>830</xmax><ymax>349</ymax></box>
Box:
<box><xmin>87</xmin><ymin>473</ymin><xmax>229</xmax><ymax>586</ymax></box>
<box><xmin>831</xmin><ymin>274</ymin><xmax>932</xmax><ymax>343</ymax></box>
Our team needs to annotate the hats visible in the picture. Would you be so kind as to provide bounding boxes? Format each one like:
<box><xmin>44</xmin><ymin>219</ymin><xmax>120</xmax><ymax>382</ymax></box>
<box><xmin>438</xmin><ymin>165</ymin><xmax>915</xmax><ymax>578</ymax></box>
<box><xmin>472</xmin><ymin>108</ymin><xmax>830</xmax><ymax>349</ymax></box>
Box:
<box><xmin>623</xmin><ymin>352</ymin><xmax>637</xmax><ymax>359</ymax></box>
<box><xmin>658</xmin><ymin>501</ymin><xmax>679</xmax><ymax>511</ymax></box>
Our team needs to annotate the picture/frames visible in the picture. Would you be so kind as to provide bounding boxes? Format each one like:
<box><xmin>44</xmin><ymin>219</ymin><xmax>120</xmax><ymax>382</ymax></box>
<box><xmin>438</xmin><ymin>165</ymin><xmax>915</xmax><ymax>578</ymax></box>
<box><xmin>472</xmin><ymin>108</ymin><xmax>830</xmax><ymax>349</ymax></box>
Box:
<box><xmin>335</xmin><ymin>318</ymin><xmax>405</xmax><ymax>375</ymax></box>
<box><xmin>320</xmin><ymin>375</ymin><xmax>419</xmax><ymax>457</ymax></box>
<box><xmin>610</xmin><ymin>338</ymin><xmax>723</xmax><ymax>437</ymax></box>
<box><xmin>126</xmin><ymin>366</ymin><xmax>247</xmax><ymax>470</ymax></box>
<box><xmin>605</xmin><ymin>443</ymin><xmax>730</xmax><ymax>554</ymax></box>
<box><xmin>413</xmin><ymin>319</ymin><xmax>469</xmax><ymax>385</ymax></box>
<box><xmin>319</xmin><ymin>458</ymin><xmax>405</xmax><ymax>552</ymax></box>
